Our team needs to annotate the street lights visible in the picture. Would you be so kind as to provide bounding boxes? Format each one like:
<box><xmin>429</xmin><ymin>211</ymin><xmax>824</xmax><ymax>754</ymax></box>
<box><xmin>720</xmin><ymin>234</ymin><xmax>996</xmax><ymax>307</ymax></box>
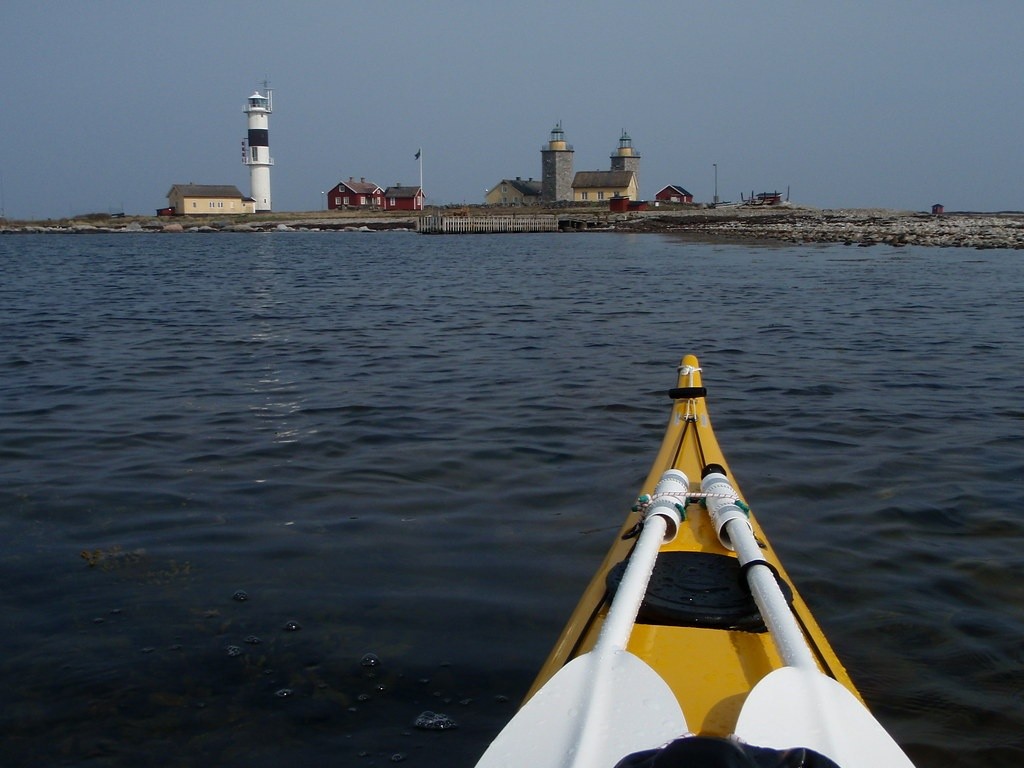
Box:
<box><xmin>713</xmin><ymin>163</ymin><xmax>717</xmax><ymax>196</ymax></box>
<box><xmin>321</xmin><ymin>191</ymin><xmax>324</xmax><ymax>209</ymax></box>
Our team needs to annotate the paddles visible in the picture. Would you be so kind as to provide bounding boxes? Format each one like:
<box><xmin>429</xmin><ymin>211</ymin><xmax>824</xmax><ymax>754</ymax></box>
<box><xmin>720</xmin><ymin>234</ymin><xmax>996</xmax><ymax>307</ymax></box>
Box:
<box><xmin>699</xmin><ymin>462</ymin><xmax>918</xmax><ymax>768</ymax></box>
<box><xmin>473</xmin><ymin>467</ymin><xmax>690</xmax><ymax>768</ymax></box>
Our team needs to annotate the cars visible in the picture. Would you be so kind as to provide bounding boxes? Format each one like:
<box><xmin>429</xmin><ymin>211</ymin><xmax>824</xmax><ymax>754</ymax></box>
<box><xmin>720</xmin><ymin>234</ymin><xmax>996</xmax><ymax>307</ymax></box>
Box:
<box><xmin>111</xmin><ymin>214</ymin><xmax>119</xmax><ymax>217</ymax></box>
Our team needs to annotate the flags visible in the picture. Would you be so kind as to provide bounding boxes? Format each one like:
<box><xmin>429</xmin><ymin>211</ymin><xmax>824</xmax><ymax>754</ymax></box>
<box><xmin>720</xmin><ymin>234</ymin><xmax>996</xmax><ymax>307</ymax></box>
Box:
<box><xmin>415</xmin><ymin>148</ymin><xmax>422</xmax><ymax>160</ymax></box>
